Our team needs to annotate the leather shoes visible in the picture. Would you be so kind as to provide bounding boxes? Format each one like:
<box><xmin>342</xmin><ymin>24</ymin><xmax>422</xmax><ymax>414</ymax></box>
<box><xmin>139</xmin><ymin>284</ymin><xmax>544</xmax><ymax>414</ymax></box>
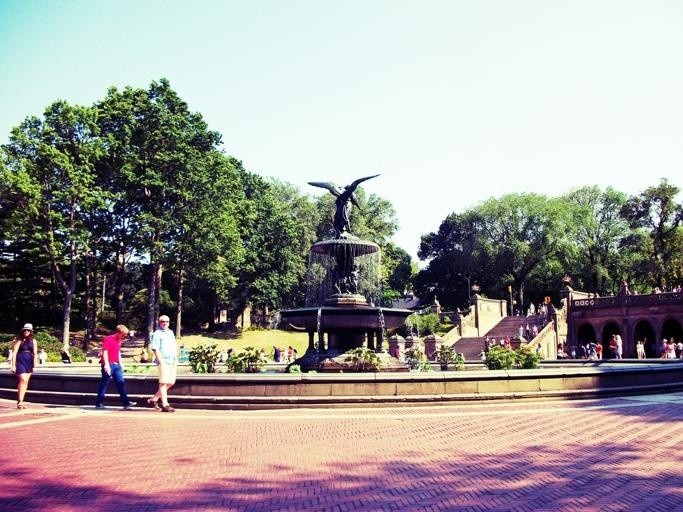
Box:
<box><xmin>96</xmin><ymin>401</ymin><xmax>106</xmax><ymax>409</ymax></box>
<box><xmin>123</xmin><ymin>400</ymin><xmax>138</xmax><ymax>408</ymax></box>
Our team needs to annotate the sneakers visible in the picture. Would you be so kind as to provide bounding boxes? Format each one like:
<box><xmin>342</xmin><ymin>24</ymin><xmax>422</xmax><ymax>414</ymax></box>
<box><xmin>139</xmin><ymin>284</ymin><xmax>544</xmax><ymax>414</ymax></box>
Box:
<box><xmin>148</xmin><ymin>397</ymin><xmax>176</xmax><ymax>413</ymax></box>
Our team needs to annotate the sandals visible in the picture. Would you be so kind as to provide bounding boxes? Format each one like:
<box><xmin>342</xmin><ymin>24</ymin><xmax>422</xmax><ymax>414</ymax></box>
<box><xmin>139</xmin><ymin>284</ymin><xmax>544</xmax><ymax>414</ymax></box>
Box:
<box><xmin>17</xmin><ymin>400</ymin><xmax>29</xmax><ymax>409</ymax></box>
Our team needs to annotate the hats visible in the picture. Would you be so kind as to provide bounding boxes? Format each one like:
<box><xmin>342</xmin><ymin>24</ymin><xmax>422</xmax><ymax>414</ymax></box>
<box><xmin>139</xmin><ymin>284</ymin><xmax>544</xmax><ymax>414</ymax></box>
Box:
<box><xmin>21</xmin><ymin>323</ymin><xmax>35</xmax><ymax>332</ymax></box>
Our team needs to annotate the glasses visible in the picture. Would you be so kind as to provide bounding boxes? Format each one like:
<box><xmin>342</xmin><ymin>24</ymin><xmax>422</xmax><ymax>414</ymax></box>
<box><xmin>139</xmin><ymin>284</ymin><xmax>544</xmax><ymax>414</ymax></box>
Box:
<box><xmin>161</xmin><ymin>321</ymin><xmax>170</xmax><ymax>323</ymax></box>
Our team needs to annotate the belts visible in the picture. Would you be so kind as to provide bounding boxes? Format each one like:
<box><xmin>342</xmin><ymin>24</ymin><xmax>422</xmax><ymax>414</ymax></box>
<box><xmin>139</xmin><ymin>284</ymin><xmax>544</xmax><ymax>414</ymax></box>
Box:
<box><xmin>110</xmin><ymin>361</ymin><xmax>119</xmax><ymax>366</ymax></box>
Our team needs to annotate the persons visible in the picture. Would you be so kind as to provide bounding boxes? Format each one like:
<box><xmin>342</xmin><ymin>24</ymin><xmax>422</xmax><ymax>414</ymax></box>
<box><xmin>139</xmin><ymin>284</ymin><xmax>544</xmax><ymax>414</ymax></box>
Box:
<box><xmin>7</xmin><ymin>349</ymin><xmax>13</xmax><ymax>364</ymax></box>
<box><xmin>392</xmin><ymin>346</ymin><xmax>400</xmax><ymax>360</ymax></box>
<box><xmin>93</xmin><ymin>323</ymin><xmax>137</xmax><ymax>411</ymax></box>
<box><xmin>10</xmin><ymin>322</ymin><xmax>38</xmax><ymax>411</ymax></box>
<box><xmin>483</xmin><ymin>336</ymin><xmax>489</xmax><ymax>350</ymax></box>
<box><xmin>176</xmin><ymin>344</ymin><xmax>298</xmax><ymax>366</ymax></box>
<box><xmin>477</xmin><ymin>349</ymin><xmax>486</xmax><ymax>361</ymax></box>
<box><xmin>127</xmin><ymin>328</ymin><xmax>135</xmax><ymax>345</ymax></box>
<box><xmin>59</xmin><ymin>347</ymin><xmax>72</xmax><ymax>365</ymax></box>
<box><xmin>514</xmin><ymin>301</ymin><xmax>548</xmax><ymax>319</ymax></box>
<box><xmin>524</xmin><ymin>323</ymin><xmax>530</xmax><ymax>338</ymax></box>
<box><xmin>555</xmin><ymin>334</ymin><xmax>682</xmax><ymax>360</ymax></box>
<box><xmin>649</xmin><ymin>283</ymin><xmax>682</xmax><ymax>295</ymax></box>
<box><xmin>498</xmin><ymin>337</ymin><xmax>504</xmax><ymax>348</ymax></box>
<box><xmin>402</xmin><ymin>346</ymin><xmax>412</xmax><ymax>364</ymax></box>
<box><xmin>126</xmin><ymin>328</ymin><xmax>132</xmax><ymax>344</ymax></box>
<box><xmin>340</xmin><ymin>184</ymin><xmax>362</xmax><ymax>233</ymax></box>
<box><xmin>145</xmin><ymin>314</ymin><xmax>179</xmax><ymax>414</ymax></box>
<box><xmin>490</xmin><ymin>336</ymin><xmax>495</xmax><ymax>344</ymax></box>
<box><xmin>38</xmin><ymin>348</ymin><xmax>47</xmax><ymax>364</ymax></box>
<box><xmin>138</xmin><ymin>348</ymin><xmax>148</xmax><ymax>363</ymax></box>
<box><xmin>503</xmin><ymin>336</ymin><xmax>510</xmax><ymax>349</ymax></box>
<box><xmin>530</xmin><ymin>324</ymin><xmax>537</xmax><ymax>338</ymax></box>
<box><xmin>518</xmin><ymin>325</ymin><xmax>523</xmax><ymax>337</ymax></box>
